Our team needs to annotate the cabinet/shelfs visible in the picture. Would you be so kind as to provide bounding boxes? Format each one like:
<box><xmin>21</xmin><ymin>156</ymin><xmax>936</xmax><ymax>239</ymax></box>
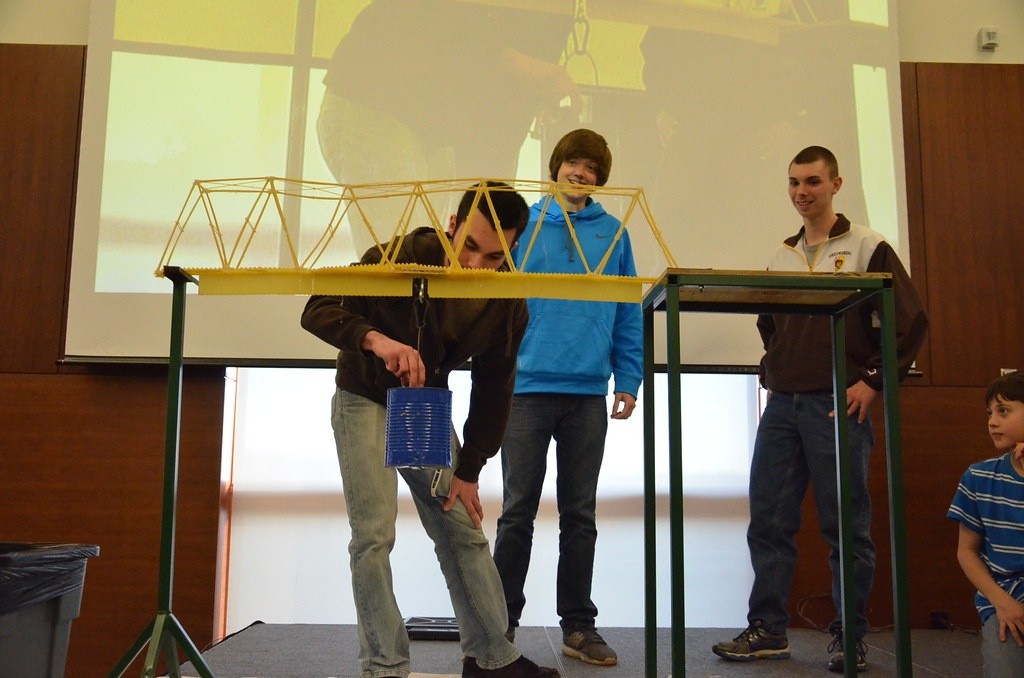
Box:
<box><xmin>787</xmin><ymin>63</ymin><xmax>1024</xmax><ymax>630</ymax></box>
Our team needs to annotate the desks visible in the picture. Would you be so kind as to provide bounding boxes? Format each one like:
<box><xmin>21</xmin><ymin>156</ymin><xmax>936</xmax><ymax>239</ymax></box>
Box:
<box><xmin>642</xmin><ymin>266</ymin><xmax>914</xmax><ymax>678</ymax></box>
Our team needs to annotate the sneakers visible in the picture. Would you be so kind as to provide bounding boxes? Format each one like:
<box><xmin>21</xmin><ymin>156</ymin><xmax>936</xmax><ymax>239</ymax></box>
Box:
<box><xmin>712</xmin><ymin>618</ymin><xmax>791</xmax><ymax>662</ymax></box>
<box><xmin>503</xmin><ymin>628</ymin><xmax>516</xmax><ymax>644</ymax></box>
<box><xmin>827</xmin><ymin>626</ymin><xmax>870</xmax><ymax>670</ymax></box>
<box><xmin>560</xmin><ymin>619</ymin><xmax>617</xmax><ymax>666</ymax></box>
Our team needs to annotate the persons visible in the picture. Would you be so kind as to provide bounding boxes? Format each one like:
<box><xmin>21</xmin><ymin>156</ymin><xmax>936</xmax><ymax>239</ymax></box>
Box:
<box><xmin>316</xmin><ymin>0</ymin><xmax>583</xmax><ymax>261</ymax></box>
<box><xmin>492</xmin><ymin>128</ymin><xmax>644</xmax><ymax>664</ymax></box>
<box><xmin>947</xmin><ymin>371</ymin><xmax>1024</xmax><ymax>678</ymax></box>
<box><xmin>709</xmin><ymin>145</ymin><xmax>930</xmax><ymax>672</ymax></box>
<box><xmin>300</xmin><ymin>180</ymin><xmax>560</xmax><ymax>678</ymax></box>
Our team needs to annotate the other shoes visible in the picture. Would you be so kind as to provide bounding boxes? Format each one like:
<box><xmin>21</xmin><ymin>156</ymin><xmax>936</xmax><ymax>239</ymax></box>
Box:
<box><xmin>462</xmin><ymin>655</ymin><xmax>561</xmax><ymax>678</ymax></box>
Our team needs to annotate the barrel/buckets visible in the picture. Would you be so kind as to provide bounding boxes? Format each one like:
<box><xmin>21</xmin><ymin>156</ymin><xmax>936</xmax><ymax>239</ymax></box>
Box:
<box><xmin>384</xmin><ymin>386</ymin><xmax>453</xmax><ymax>470</ymax></box>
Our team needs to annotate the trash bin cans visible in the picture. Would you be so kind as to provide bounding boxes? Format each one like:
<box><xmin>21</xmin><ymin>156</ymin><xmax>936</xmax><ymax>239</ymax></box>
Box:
<box><xmin>1</xmin><ymin>544</ymin><xmax>100</xmax><ymax>677</ymax></box>
